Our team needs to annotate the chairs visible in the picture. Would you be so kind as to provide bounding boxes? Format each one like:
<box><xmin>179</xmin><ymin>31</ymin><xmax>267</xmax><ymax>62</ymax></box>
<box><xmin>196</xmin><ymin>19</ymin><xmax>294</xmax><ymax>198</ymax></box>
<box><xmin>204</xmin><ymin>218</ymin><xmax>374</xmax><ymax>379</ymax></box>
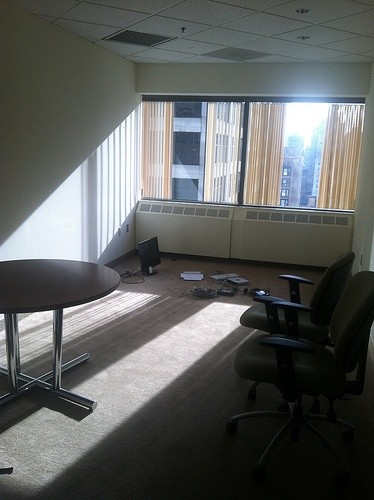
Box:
<box><xmin>226</xmin><ymin>251</ymin><xmax>374</xmax><ymax>487</ymax></box>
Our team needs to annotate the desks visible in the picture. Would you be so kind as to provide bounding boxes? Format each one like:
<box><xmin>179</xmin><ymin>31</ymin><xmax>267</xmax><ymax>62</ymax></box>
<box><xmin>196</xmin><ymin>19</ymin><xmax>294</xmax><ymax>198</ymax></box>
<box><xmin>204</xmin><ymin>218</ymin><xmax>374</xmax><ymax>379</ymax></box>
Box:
<box><xmin>0</xmin><ymin>259</ymin><xmax>120</xmax><ymax>411</ymax></box>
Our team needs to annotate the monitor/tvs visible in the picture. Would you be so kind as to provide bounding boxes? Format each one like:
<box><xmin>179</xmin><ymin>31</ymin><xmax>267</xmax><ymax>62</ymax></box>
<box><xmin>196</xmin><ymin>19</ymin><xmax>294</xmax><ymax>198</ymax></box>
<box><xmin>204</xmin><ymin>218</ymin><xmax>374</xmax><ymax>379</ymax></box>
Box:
<box><xmin>138</xmin><ymin>236</ymin><xmax>161</xmax><ymax>276</ymax></box>
